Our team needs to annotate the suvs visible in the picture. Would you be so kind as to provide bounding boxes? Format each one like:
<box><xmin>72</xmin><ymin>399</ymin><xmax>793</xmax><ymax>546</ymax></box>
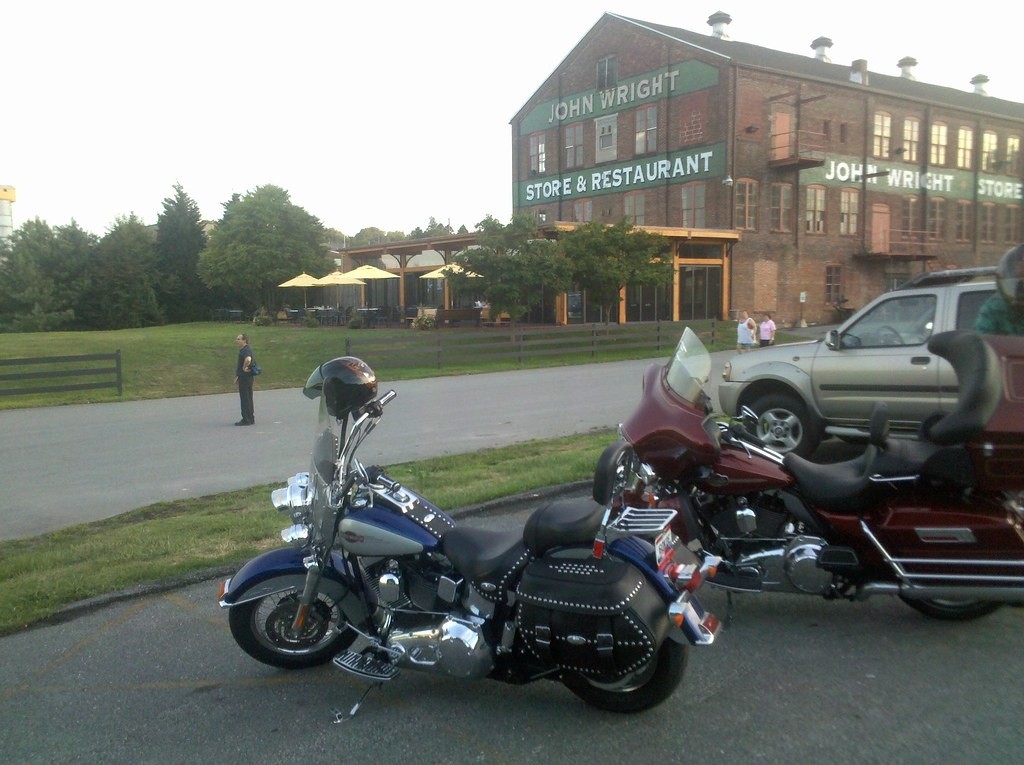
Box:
<box><xmin>717</xmin><ymin>266</ymin><xmax>1024</xmax><ymax>461</ymax></box>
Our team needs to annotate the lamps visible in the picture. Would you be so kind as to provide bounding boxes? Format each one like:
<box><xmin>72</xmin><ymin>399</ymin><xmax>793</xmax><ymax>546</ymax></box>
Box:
<box><xmin>745</xmin><ymin>124</ymin><xmax>760</xmax><ymax>134</ymax></box>
<box><xmin>529</xmin><ymin>170</ymin><xmax>542</xmax><ymax>178</ymax></box>
<box><xmin>999</xmin><ymin>161</ymin><xmax>1012</xmax><ymax>168</ymax></box>
<box><xmin>894</xmin><ymin>147</ymin><xmax>909</xmax><ymax>155</ymax></box>
<box><xmin>721</xmin><ymin>173</ymin><xmax>733</xmax><ymax>186</ymax></box>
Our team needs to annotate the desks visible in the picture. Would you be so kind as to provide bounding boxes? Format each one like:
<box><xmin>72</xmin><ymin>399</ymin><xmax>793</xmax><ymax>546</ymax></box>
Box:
<box><xmin>408</xmin><ymin>307</ymin><xmax>434</xmax><ymax>310</ymax></box>
<box><xmin>307</xmin><ymin>307</ymin><xmax>333</xmax><ymax>327</ymax></box>
<box><xmin>357</xmin><ymin>308</ymin><xmax>380</xmax><ymax>328</ymax></box>
<box><xmin>288</xmin><ymin>310</ymin><xmax>299</xmax><ymax>324</ymax></box>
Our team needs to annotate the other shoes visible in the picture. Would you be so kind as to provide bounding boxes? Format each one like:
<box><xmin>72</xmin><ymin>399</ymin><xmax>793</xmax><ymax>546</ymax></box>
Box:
<box><xmin>235</xmin><ymin>419</ymin><xmax>254</xmax><ymax>425</ymax></box>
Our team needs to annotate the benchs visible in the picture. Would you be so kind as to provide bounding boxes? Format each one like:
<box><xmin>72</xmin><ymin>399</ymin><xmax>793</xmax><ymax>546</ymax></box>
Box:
<box><xmin>436</xmin><ymin>308</ymin><xmax>484</xmax><ymax>327</ymax></box>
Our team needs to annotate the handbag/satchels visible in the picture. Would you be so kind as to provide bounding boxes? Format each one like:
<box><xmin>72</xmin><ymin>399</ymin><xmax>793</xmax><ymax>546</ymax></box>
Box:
<box><xmin>250</xmin><ymin>366</ymin><xmax>261</xmax><ymax>376</ymax></box>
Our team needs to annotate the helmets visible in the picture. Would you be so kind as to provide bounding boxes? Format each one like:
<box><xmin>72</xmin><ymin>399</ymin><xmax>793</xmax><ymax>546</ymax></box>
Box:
<box><xmin>997</xmin><ymin>244</ymin><xmax>1024</xmax><ymax>307</ymax></box>
<box><xmin>315</xmin><ymin>356</ymin><xmax>377</xmax><ymax>416</ymax></box>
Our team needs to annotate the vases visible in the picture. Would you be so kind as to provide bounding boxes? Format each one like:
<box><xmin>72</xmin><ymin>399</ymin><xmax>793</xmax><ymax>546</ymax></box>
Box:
<box><xmin>419</xmin><ymin>323</ymin><xmax>425</xmax><ymax>330</ymax></box>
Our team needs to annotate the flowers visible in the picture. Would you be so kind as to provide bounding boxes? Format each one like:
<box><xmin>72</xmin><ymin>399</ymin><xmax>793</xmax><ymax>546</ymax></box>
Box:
<box><xmin>409</xmin><ymin>314</ymin><xmax>435</xmax><ymax>328</ymax></box>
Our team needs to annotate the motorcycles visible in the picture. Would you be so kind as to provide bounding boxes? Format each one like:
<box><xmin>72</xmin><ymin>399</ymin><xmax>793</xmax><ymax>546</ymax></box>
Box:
<box><xmin>608</xmin><ymin>325</ymin><xmax>1024</xmax><ymax>623</ymax></box>
<box><xmin>217</xmin><ymin>387</ymin><xmax>725</xmax><ymax>724</ymax></box>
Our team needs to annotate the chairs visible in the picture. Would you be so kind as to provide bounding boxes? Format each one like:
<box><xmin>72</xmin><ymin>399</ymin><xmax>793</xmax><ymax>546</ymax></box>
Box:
<box><xmin>277</xmin><ymin>304</ymin><xmax>444</xmax><ymax>328</ymax></box>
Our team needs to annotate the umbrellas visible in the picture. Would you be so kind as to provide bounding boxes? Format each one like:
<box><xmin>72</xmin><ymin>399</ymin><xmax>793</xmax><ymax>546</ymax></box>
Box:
<box><xmin>277</xmin><ymin>262</ymin><xmax>402</xmax><ymax>319</ymax></box>
<box><xmin>419</xmin><ymin>264</ymin><xmax>485</xmax><ymax>323</ymax></box>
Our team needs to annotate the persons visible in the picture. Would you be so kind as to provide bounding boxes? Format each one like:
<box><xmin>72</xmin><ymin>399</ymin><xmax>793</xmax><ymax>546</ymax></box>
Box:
<box><xmin>759</xmin><ymin>313</ymin><xmax>776</xmax><ymax>346</ymax></box>
<box><xmin>280</xmin><ymin>303</ymin><xmax>286</xmax><ymax>311</ymax></box>
<box><xmin>737</xmin><ymin>310</ymin><xmax>757</xmax><ymax>354</ymax></box>
<box><xmin>972</xmin><ymin>243</ymin><xmax>1024</xmax><ymax>336</ymax></box>
<box><xmin>233</xmin><ymin>334</ymin><xmax>255</xmax><ymax>427</ymax></box>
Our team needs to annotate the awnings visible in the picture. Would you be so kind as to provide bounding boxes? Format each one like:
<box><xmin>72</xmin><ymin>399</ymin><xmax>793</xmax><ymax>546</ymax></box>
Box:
<box><xmin>769</xmin><ymin>157</ymin><xmax>825</xmax><ymax>172</ymax></box>
<box><xmin>852</xmin><ymin>251</ymin><xmax>938</xmax><ymax>265</ymax></box>
